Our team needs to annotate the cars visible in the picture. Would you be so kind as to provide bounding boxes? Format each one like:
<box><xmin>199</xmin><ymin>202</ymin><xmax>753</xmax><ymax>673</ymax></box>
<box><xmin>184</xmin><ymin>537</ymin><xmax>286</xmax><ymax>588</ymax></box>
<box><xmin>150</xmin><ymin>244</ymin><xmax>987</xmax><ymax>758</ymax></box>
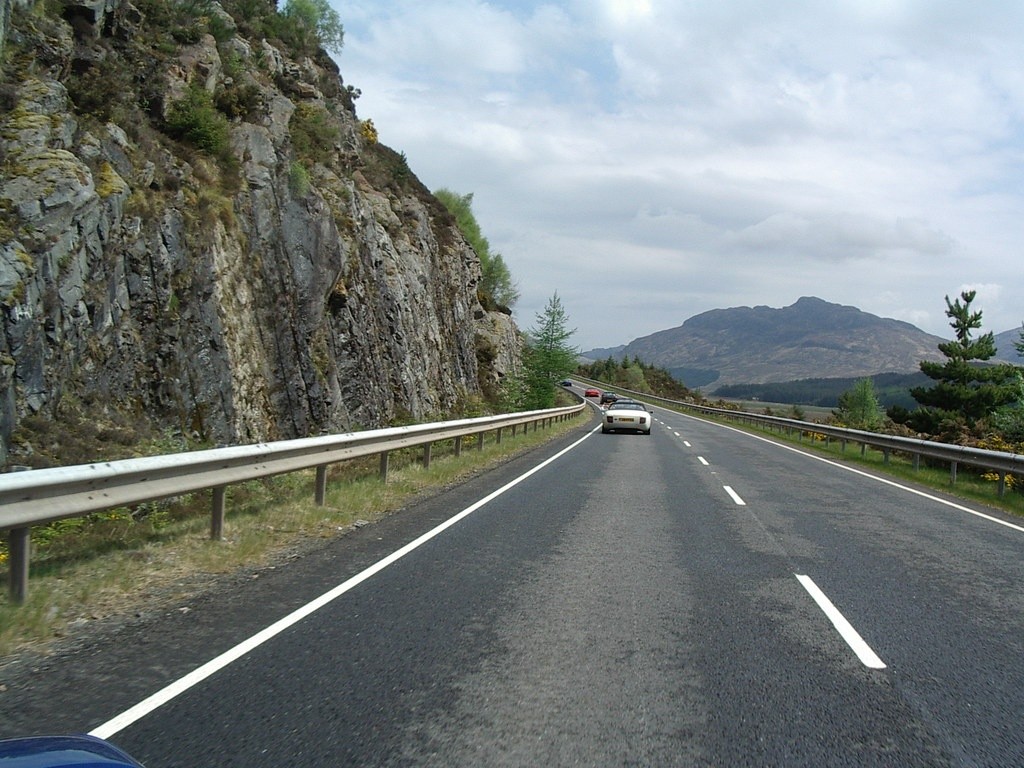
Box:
<box><xmin>561</xmin><ymin>379</ymin><xmax>571</xmax><ymax>387</ymax></box>
<box><xmin>585</xmin><ymin>388</ymin><xmax>600</xmax><ymax>397</ymax></box>
<box><xmin>600</xmin><ymin>391</ymin><xmax>616</xmax><ymax>404</ymax></box>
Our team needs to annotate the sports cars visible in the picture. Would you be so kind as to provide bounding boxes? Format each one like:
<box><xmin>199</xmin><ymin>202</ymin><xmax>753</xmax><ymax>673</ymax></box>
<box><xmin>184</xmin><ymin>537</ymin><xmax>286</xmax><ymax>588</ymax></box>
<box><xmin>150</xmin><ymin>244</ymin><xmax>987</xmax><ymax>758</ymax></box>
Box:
<box><xmin>602</xmin><ymin>399</ymin><xmax>654</xmax><ymax>435</ymax></box>
